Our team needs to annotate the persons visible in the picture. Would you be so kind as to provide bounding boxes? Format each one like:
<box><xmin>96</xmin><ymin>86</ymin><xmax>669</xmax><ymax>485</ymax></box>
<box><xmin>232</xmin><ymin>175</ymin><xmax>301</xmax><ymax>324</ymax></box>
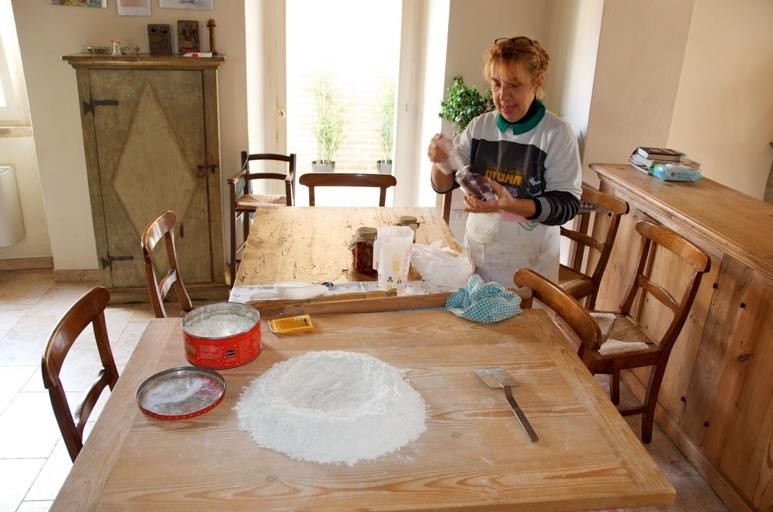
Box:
<box><xmin>428</xmin><ymin>35</ymin><xmax>583</xmax><ymax>318</ymax></box>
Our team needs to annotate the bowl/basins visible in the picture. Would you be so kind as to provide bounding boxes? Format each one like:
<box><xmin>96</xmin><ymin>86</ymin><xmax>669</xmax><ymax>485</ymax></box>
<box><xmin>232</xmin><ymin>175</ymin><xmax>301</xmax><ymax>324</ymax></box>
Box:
<box><xmin>85</xmin><ymin>46</ymin><xmax>109</xmax><ymax>54</ymax></box>
<box><xmin>180</xmin><ymin>302</ymin><xmax>262</xmax><ymax>370</ymax></box>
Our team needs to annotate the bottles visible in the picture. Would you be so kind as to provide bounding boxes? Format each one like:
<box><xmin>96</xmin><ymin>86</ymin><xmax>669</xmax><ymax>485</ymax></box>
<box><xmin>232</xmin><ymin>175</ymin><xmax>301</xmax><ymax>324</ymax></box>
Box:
<box><xmin>352</xmin><ymin>225</ymin><xmax>377</xmax><ymax>274</ymax></box>
<box><xmin>110</xmin><ymin>40</ymin><xmax>121</xmax><ymax>56</ymax></box>
<box><xmin>399</xmin><ymin>216</ymin><xmax>420</xmax><ymax>239</ymax></box>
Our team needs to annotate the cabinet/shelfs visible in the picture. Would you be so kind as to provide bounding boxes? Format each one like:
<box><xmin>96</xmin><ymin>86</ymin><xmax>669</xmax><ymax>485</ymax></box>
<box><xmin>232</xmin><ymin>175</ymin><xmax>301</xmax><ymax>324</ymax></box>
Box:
<box><xmin>586</xmin><ymin>162</ymin><xmax>772</xmax><ymax>512</ymax></box>
<box><xmin>61</xmin><ymin>51</ymin><xmax>231</xmax><ymax>309</ymax></box>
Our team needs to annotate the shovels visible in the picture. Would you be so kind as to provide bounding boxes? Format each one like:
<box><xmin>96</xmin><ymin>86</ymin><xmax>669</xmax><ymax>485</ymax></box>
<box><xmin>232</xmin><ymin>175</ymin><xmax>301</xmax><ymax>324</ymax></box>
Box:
<box><xmin>472</xmin><ymin>368</ymin><xmax>539</xmax><ymax>443</ymax></box>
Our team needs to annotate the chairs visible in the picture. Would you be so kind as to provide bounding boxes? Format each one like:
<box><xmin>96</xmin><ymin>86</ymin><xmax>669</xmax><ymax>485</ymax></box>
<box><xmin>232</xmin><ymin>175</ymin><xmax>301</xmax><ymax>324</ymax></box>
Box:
<box><xmin>227</xmin><ymin>151</ymin><xmax>298</xmax><ymax>284</ymax></box>
<box><xmin>298</xmin><ymin>171</ymin><xmax>398</xmax><ymax>207</ymax></box>
<box><xmin>41</xmin><ymin>284</ymin><xmax>117</xmax><ymax>463</ymax></box>
<box><xmin>585</xmin><ymin>219</ymin><xmax>711</xmax><ymax>445</ymax></box>
<box><xmin>558</xmin><ymin>180</ymin><xmax>628</xmax><ymax>312</ymax></box>
<box><xmin>138</xmin><ymin>209</ymin><xmax>194</xmax><ymax>320</ymax></box>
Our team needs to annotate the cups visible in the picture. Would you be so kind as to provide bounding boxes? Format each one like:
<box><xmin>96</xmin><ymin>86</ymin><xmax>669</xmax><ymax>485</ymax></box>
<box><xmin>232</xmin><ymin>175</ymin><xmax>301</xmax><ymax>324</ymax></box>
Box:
<box><xmin>371</xmin><ymin>225</ymin><xmax>415</xmax><ymax>284</ymax></box>
<box><xmin>454</xmin><ymin>164</ymin><xmax>496</xmax><ymax>206</ymax></box>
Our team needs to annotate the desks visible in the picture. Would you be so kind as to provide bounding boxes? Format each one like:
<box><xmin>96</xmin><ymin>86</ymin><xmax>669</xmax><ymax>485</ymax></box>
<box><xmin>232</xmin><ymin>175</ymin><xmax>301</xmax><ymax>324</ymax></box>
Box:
<box><xmin>226</xmin><ymin>205</ymin><xmax>467</xmax><ymax>321</ymax></box>
<box><xmin>42</xmin><ymin>322</ymin><xmax>682</xmax><ymax>512</ymax></box>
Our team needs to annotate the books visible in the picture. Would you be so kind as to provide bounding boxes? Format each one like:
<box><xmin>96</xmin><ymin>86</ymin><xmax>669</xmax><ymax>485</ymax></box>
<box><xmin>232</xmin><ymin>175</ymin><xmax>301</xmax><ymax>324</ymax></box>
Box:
<box><xmin>629</xmin><ymin>146</ymin><xmax>686</xmax><ymax>175</ymax></box>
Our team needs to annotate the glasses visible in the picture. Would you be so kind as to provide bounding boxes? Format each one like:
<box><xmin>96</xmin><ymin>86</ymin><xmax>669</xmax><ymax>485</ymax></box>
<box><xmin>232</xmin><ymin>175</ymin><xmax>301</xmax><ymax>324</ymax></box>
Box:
<box><xmin>495</xmin><ymin>37</ymin><xmax>537</xmax><ymax>47</ymax></box>
